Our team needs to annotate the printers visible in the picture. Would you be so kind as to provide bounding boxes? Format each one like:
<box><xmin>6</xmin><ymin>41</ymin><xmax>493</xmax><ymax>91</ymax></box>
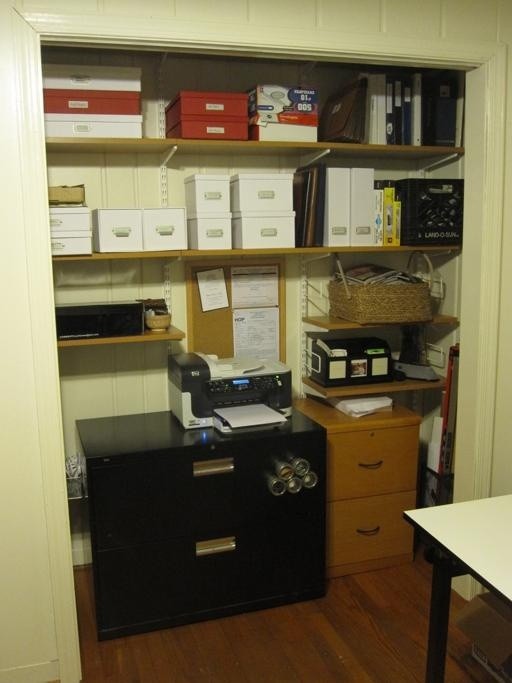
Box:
<box><xmin>168</xmin><ymin>352</ymin><xmax>293</xmax><ymax>434</ymax></box>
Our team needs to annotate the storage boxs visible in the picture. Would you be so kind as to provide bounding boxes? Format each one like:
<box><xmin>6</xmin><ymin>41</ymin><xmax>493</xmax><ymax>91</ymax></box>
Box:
<box><xmin>50</xmin><ymin>173</ymin><xmax>298</xmax><ymax>257</ymax></box>
<box><xmin>43</xmin><ymin>63</ymin><xmax>320</xmax><ymax>143</ymax></box>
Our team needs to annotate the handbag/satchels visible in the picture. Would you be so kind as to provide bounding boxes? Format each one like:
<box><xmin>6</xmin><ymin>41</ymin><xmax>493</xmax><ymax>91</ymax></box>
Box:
<box><xmin>318</xmin><ymin>78</ymin><xmax>369</xmax><ymax>143</ymax></box>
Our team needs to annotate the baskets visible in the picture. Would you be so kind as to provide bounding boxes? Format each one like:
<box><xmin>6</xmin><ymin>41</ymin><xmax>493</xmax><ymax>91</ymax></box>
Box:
<box><xmin>396</xmin><ymin>177</ymin><xmax>464</xmax><ymax>245</ymax></box>
<box><xmin>326</xmin><ymin>250</ymin><xmax>434</xmax><ymax>325</ymax></box>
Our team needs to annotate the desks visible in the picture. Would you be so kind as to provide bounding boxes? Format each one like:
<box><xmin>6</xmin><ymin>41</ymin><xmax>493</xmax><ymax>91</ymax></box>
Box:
<box><xmin>402</xmin><ymin>494</ymin><xmax>512</xmax><ymax>682</ymax></box>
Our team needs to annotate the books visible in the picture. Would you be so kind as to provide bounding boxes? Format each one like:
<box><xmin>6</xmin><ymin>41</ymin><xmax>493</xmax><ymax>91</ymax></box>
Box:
<box><xmin>336</xmin><ymin>261</ymin><xmax>426</xmax><ymax>285</ymax></box>
<box><xmin>296</xmin><ymin>167</ymin><xmax>318</xmax><ymax>247</ymax></box>
<box><xmin>293</xmin><ymin>172</ymin><xmax>311</xmax><ymax>247</ymax></box>
<box><xmin>309</xmin><ymin>163</ymin><xmax>326</xmax><ymax>247</ymax></box>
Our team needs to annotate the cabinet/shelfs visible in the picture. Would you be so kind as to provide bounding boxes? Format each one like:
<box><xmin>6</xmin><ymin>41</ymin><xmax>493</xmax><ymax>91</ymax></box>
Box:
<box><xmin>74</xmin><ymin>411</ymin><xmax>327</xmax><ymax>642</ymax></box>
<box><xmin>291</xmin><ymin>399</ymin><xmax>424</xmax><ymax>580</ymax></box>
<box><xmin>46</xmin><ymin>139</ymin><xmax>466</xmax><ymax>261</ymax></box>
<box><xmin>299</xmin><ymin>260</ymin><xmax>458</xmax><ymax>400</ymax></box>
